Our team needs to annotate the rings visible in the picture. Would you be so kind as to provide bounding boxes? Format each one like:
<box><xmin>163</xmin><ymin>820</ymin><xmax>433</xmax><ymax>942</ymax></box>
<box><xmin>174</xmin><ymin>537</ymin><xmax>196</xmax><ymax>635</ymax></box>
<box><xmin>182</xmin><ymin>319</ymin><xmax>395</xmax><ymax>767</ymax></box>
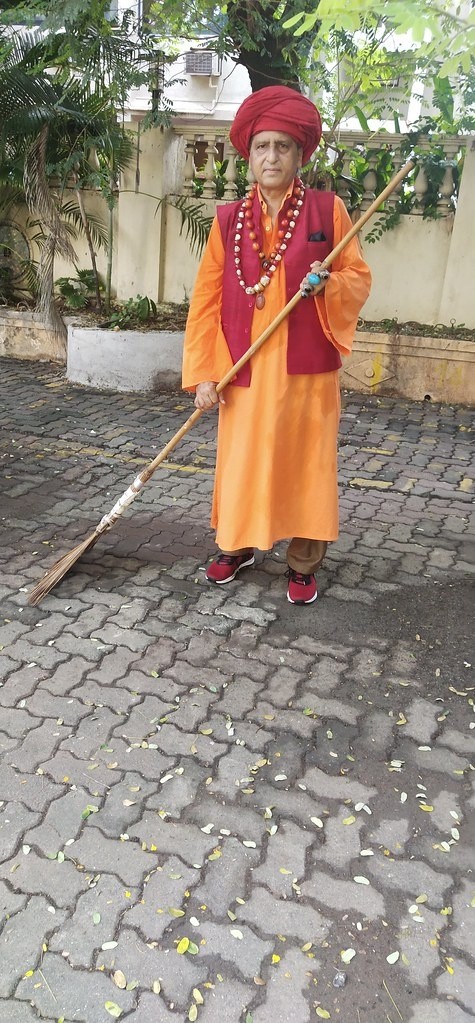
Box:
<box><xmin>300</xmin><ymin>270</ymin><xmax>330</xmax><ymax>298</ymax></box>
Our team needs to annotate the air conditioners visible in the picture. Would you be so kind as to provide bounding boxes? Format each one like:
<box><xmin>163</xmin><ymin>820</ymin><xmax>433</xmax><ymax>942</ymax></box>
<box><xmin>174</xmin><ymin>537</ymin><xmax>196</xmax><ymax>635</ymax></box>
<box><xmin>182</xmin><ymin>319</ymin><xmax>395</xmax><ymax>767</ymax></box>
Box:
<box><xmin>184</xmin><ymin>51</ymin><xmax>221</xmax><ymax>76</ymax></box>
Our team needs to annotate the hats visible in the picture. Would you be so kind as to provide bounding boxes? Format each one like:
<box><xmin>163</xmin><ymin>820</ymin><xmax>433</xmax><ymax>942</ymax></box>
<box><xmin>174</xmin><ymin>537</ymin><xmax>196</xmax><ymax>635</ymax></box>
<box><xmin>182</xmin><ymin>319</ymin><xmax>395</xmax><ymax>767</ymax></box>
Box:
<box><xmin>229</xmin><ymin>85</ymin><xmax>322</xmax><ymax>167</ymax></box>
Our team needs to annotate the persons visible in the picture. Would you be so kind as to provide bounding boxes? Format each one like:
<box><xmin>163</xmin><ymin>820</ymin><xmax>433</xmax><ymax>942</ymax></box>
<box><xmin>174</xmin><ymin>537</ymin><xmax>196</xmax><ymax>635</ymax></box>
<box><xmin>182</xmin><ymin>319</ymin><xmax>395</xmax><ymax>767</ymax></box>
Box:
<box><xmin>182</xmin><ymin>85</ymin><xmax>373</xmax><ymax>605</ymax></box>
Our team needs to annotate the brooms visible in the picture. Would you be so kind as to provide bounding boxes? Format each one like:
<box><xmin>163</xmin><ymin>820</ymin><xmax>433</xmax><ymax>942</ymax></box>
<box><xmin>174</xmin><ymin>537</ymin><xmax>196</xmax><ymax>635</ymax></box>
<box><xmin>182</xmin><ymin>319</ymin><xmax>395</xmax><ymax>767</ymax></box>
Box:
<box><xmin>24</xmin><ymin>160</ymin><xmax>417</xmax><ymax>609</ymax></box>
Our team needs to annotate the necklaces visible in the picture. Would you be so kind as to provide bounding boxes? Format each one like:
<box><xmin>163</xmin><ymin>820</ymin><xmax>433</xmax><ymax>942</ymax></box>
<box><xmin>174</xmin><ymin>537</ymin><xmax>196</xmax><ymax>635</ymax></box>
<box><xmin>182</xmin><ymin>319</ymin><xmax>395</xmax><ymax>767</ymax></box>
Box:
<box><xmin>232</xmin><ymin>178</ymin><xmax>305</xmax><ymax>295</ymax></box>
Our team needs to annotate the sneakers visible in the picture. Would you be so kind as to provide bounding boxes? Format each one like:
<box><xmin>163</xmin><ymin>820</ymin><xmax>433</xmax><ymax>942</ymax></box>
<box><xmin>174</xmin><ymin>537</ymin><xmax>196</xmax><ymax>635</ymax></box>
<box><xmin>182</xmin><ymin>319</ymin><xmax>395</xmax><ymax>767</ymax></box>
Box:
<box><xmin>284</xmin><ymin>565</ymin><xmax>318</xmax><ymax>605</ymax></box>
<box><xmin>205</xmin><ymin>550</ymin><xmax>255</xmax><ymax>584</ymax></box>
